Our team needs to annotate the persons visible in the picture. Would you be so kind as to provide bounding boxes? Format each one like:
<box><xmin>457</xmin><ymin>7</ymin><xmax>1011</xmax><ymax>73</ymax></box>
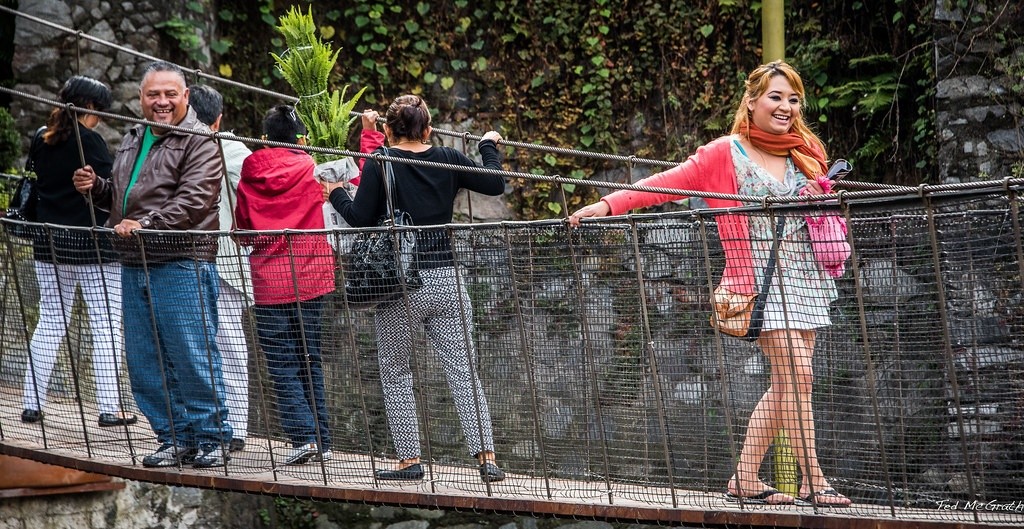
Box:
<box><xmin>321</xmin><ymin>94</ymin><xmax>508</xmax><ymax>483</ymax></box>
<box><xmin>567</xmin><ymin>58</ymin><xmax>856</xmax><ymax>509</ymax></box>
<box><xmin>227</xmin><ymin>109</ymin><xmax>387</xmax><ymax>467</ymax></box>
<box><xmin>19</xmin><ymin>77</ymin><xmax>138</xmax><ymax>428</ymax></box>
<box><xmin>184</xmin><ymin>86</ymin><xmax>254</xmax><ymax>454</ymax></box>
<box><xmin>73</xmin><ymin>61</ymin><xmax>235</xmax><ymax>471</ymax></box>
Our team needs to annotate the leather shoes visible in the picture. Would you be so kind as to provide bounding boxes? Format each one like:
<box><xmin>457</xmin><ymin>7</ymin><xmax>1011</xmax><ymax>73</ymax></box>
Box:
<box><xmin>98</xmin><ymin>413</ymin><xmax>138</xmax><ymax>427</ymax></box>
<box><xmin>22</xmin><ymin>409</ymin><xmax>44</xmax><ymax>423</ymax></box>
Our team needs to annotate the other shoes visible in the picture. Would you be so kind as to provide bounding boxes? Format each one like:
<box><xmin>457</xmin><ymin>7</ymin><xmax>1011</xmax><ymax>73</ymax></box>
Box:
<box><xmin>374</xmin><ymin>464</ymin><xmax>424</xmax><ymax>480</ymax></box>
<box><xmin>229</xmin><ymin>438</ymin><xmax>244</xmax><ymax>452</ymax></box>
<box><xmin>479</xmin><ymin>463</ymin><xmax>506</xmax><ymax>482</ymax></box>
<box><xmin>311</xmin><ymin>449</ymin><xmax>332</xmax><ymax>462</ymax></box>
<box><xmin>285</xmin><ymin>443</ymin><xmax>318</xmax><ymax>464</ymax></box>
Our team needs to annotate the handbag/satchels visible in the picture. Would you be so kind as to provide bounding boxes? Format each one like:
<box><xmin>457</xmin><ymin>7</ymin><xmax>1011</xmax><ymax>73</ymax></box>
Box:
<box><xmin>343</xmin><ymin>145</ymin><xmax>422</xmax><ymax>311</ymax></box>
<box><xmin>709</xmin><ymin>286</ymin><xmax>765</xmax><ymax>341</ymax></box>
<box><xmin>3</xmin><ymin>126</ymin><xmax>52</xmax><ymax>238</ymax></box>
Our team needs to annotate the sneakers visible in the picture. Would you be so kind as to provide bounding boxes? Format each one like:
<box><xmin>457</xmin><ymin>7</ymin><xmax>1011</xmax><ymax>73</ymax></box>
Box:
<box><xmin>143</xmin><ymin>443</ymin><xmax>200</xmax><ymax>467</ymax></box>
<box><xmin>194</xmin><ymin>442</ymin><xmax>231</xmax><ymax>467</ymax></box>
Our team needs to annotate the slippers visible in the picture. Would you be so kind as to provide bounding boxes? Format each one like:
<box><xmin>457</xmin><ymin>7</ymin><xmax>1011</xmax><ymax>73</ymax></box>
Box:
<box><xmin>794</xmin><ymin>490</ymin><xmax>849</xmax><ymax>508</ymax></box>
<box><xmin>725</xmin><ymin>488</ymin><xmax>794</xmax><ymax>505</ymax></box>
